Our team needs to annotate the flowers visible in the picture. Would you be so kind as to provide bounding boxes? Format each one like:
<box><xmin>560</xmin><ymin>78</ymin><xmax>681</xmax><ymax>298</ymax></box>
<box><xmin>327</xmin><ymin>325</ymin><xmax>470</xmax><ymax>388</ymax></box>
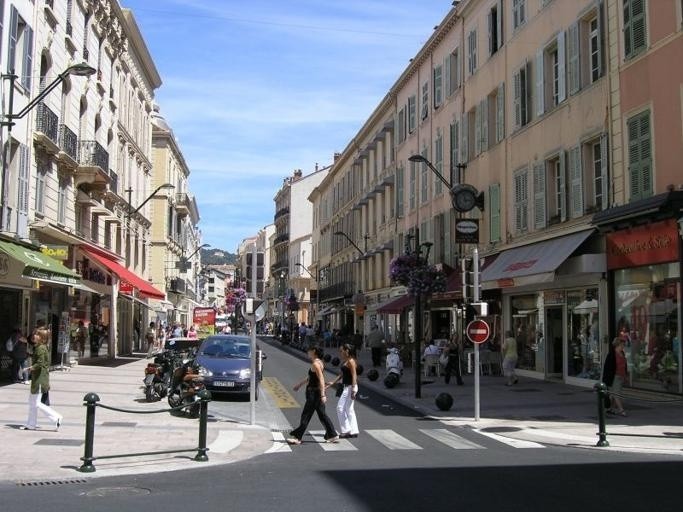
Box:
<box><xmin>386</xmin><ymin>254</ymin><xmax>428</xmax><ymax>290</ymax></box>
<box><xmin>405</xmin><ymin>265</ymin><xmax>448</xmax><ymax>302</ymax></box>
<box><xmin>282</xmin><ymin>295</ymin><xmax>300</xmax><ymax>311</ymax></box>
<box><xmin>223</xmin><ymin>288</ymin><xmax>248</xmax><ymax>315</ymax></box>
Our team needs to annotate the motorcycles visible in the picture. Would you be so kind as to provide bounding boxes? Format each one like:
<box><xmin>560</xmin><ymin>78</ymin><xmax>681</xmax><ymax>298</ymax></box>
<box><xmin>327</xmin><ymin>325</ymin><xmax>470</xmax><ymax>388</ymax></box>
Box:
<box><xmin>138</xmin><ymin>337</ymin><xmax>204</xmax><ymax>421</ymax></box>
<box><xmin>384</xmin><ymin>346</ymin><xmax>403</xmax><ymax>376</ymax></box>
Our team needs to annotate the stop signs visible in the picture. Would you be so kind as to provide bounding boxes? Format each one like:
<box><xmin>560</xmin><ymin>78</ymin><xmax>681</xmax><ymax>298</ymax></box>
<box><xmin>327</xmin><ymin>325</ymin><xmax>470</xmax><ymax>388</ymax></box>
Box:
<box><xmin>465</xmin><ymin>320</ymin><xmax>488</xmax><ymax>343</ymax></box>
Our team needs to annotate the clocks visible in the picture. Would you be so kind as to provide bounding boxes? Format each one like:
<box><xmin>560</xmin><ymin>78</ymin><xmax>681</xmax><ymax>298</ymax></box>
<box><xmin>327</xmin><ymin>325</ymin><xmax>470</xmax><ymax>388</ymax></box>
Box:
<box><xmin>453</xmin><ymin>190</ymin><xmax>476</xmax><ymax>213</ymax></box>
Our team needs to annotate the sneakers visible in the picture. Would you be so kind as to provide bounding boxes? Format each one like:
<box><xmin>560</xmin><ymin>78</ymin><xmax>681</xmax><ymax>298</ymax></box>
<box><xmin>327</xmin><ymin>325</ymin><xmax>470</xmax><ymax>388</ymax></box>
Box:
<box><xmin>286</xmin><ymin>437</ymin><xmax>301</xmax><ymax>445</ymax></box>
<box><xmin>325</xmin><ymin>435</ymin><xmax>339</xmax><ymax>443</ymax></box>
<box><xmin>54</xmin><ymin>416</ymin><xmax>64</xmax><ymax>432</ymax></box>
<box><xmin>457</xmin><ymin>382</ymin><xmax>464</xmax><ymax>385</ymax></box>
<box><xmin>504</xmin><ymin>378</ymin><xmax>519</xmax><ymax>386</ymax></box>
<box><xmin>444</xmin><ymin>378</ymin><xmax>449</xmax><ymax>384</ymax></box>
<box><xmin>19</xmin><ymin>424</ymin><xmax>36</xmax><ymax>430</ymax></box>
<box><xmin>606</xmin><ymin>408</ymin><xmax>629</xmax><ymax>418</ymax></box>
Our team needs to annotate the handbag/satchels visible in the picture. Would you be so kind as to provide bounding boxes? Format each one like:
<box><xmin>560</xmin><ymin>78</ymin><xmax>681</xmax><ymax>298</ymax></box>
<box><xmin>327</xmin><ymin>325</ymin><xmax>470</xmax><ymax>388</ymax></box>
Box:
<box><xmin>603</xmin><ymin>394</ymin><xmax>611</xmax><ymax>409</ymax></box>
<box><xmin>439</xmin><ymin>353</ymin><xmax>449</xmax><ymax>367</ymax></box>
<box><xmin>335</xmin><ymin>383</ymin><xmax>344</xmax><ymax>397</ymax></box>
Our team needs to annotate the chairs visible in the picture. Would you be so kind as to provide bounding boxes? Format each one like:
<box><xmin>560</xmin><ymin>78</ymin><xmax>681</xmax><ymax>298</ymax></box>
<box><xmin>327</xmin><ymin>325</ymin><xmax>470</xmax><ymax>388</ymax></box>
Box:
<box><xmin>378</xmin><ymin>334</ymin><xmax>499</xmax><ymax>379</ymax></box>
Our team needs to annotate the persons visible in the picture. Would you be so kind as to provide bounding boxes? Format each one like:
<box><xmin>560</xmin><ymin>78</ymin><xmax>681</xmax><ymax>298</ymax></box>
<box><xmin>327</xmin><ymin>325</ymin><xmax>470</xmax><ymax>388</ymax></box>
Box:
<box><xmin>440</xmin><ymin>331</ymin><xmax>464</xmax><ymax>387</ymax></box>
<box><xmin>31</xmin><ymin>319</ymin><xmax>50</xmax><ymax>350</ymax></box>
<box><xmin>324</xmin><ymin>343</ymin><xmax>358</xmax><ymax>438</ymax></box>
<box><xmin>598</xmin><ymin>336</ymin><xmax>628</xmax><ymax>418</ymax></box>
<box><xmin>9</xmin><ymin>323</ymin><xmax>28</xmax><ymax>385</ymax></box>
<box><xmin>144</xmin><ymin>320</ymin><xmax>196</xmax><ymax>356</ymax></box>
<box><xmin>365</xmin><ymin>324</ymin><xmax>384</xmax><ymax>367</ymax></box>
<box><xmin>73</xmin><ymin>321</ymin><xmax>86</xmax><ymax>358</ymax></box>
<box><xmin>285</xmin><ymin>343</ymin><xmax>338</xmax><ymax>447</ymax></box>
<box><xmin>222</xmin><ymin>324</ymin><xmax>230</xmax><ymax>335</ymax></box>
<box><xmin>18</xmin><ymin>327</ymin><xmax>62</xmax><ymax>431</ymax></box>
<box><xmin>501</xmin><ymin>330</ymin><xmax>519</xmax><ymax>384</ymax></box>
<box><xmin>298</xmin><ymin>321</ymin><xmax>362</xmax><ymax>350</ymax></box>
<box><xmin>423</xmin><ymin>337</ymin><xmax>440</xmax><ymax>358</ymax></box>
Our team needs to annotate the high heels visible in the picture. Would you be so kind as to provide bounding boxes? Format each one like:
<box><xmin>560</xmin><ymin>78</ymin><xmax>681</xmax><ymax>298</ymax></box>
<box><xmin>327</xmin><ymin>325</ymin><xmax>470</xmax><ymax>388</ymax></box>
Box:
<box><xmin>339</xmin><ymin>432</ymin><xmax>358</xmax><ymax>438</ymax></box>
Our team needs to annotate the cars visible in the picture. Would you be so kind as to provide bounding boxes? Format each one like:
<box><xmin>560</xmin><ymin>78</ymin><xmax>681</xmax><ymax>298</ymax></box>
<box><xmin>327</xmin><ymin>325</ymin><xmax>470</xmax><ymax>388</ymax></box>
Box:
<box><xmin>373</xmin><ymin>322</ymin><xmax>412</xmax><ymax>342</ymax></box>
<box><xmin>65</xmin><ymin>324</ymin><xmax>102</xmax><ymax>352</ymax></box>
<box><xmin>214</xmin><ymin>315</ymin><xmax>244</xmax><ymax>334</ymax></box>
<box><xmin>188</xmin><ymin>333</ymin><xmax>268</xmax><ymax>402</ymax></box>
